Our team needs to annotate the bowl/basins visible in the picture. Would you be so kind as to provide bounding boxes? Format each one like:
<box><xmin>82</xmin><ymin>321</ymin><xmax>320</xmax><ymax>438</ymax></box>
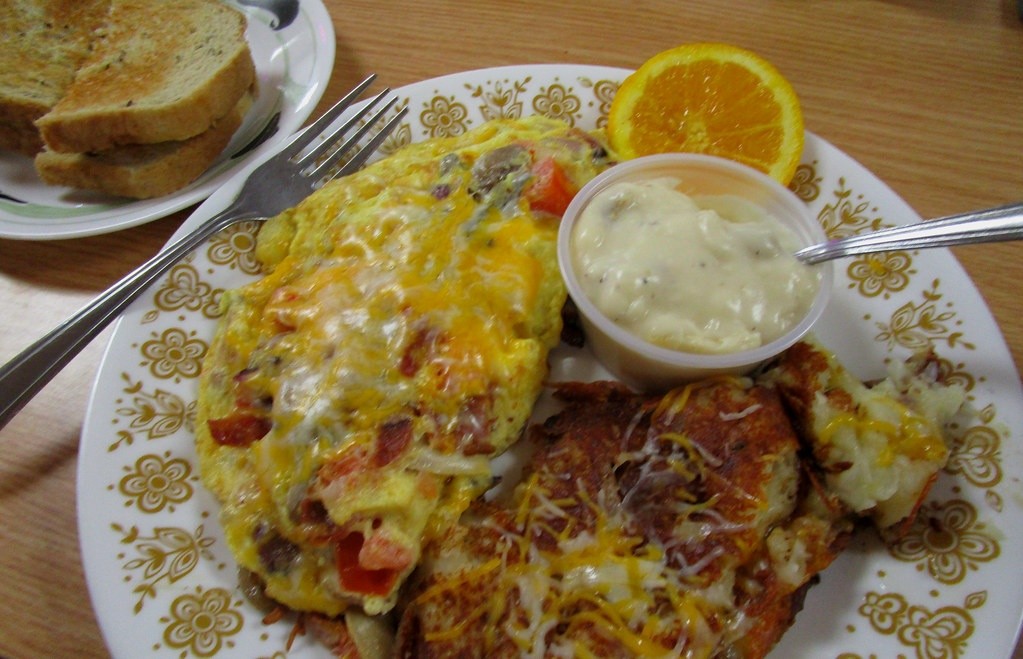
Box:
<box><xmin>557</xmin><ymin>154</ymin><xmax>836</xmax><ymax>395</ymax></box>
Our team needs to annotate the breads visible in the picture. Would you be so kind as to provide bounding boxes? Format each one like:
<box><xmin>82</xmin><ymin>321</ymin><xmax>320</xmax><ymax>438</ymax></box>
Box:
<box><xmin>0</xmin><ymin>0</ymin><xmax>259</xmax><ymax>197</ymax></box>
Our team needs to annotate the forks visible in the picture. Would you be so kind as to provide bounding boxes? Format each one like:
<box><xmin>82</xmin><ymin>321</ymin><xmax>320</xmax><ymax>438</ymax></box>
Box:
<box><xmin>0</xmin><ymin>73</ymin><xmax>411</xmax><ymax>430</ymax></box>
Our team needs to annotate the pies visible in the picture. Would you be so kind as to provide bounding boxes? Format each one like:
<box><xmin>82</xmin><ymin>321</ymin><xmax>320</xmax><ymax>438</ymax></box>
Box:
<box><xmin>187</xmin><ymin>116</ymin><xmax>610</xmax><ymax>615</ymax></box>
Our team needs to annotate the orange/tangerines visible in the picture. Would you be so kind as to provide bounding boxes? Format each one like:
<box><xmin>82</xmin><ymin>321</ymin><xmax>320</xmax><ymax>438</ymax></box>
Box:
<box><xmin>603</xmin><ymin>43</ymin><xmax>805</xmax><ymax>194</ymax></box>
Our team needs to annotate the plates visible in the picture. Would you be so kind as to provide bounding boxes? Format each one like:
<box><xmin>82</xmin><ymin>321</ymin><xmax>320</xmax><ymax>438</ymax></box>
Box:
<box><xmin>76</xmin><ymin>63</ymin><xmax>1023</xmax><ymax>659</ymax></box>
<box><xmin>0</xmin><ymin>0</ymin><xmax>336</xmax><ymax>234</ymax></box>
